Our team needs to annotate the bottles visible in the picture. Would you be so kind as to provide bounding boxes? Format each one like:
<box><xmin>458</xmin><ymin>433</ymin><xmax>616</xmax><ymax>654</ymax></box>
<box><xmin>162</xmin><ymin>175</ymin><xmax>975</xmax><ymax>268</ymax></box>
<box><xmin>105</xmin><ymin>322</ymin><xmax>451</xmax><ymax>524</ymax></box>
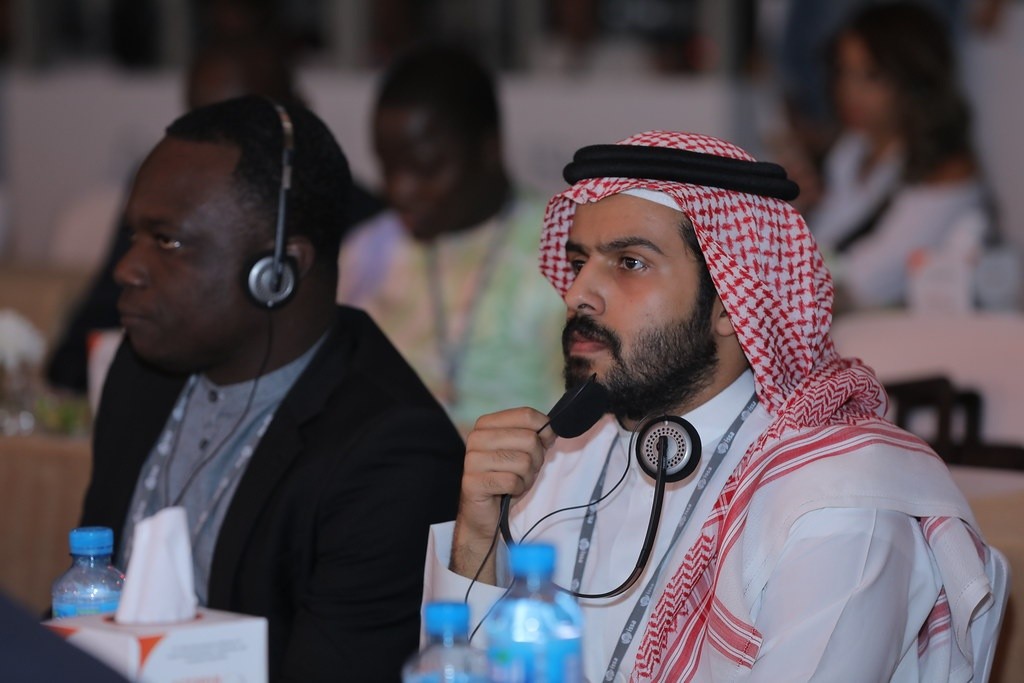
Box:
<box><xmin>484</xmin><ymin>543</ymin><xmax>586</xmax><ymax>683</ymax></box>
<box><xmin>51</xmin><ymin>527</ymin><xmax>126</xmax><ymax>618</ymax></box>
<box><xmin>402</xmin><ymin>600</ymin><xmax>491</xmax><ymax>682</ymax></box>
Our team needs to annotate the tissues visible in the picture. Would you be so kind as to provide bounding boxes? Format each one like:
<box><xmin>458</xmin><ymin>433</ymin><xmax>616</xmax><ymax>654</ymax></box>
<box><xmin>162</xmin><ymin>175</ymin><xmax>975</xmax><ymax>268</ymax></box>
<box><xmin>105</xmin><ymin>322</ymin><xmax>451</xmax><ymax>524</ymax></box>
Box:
<box><xmin>40</xmin><ymin>507</ymin><xmax>268</xmax><ymax>683</ymax></box>
<box><xmin>906</xmin><ymin>202</ymin><xmax>990</xmax><ymax>312</ymax></box>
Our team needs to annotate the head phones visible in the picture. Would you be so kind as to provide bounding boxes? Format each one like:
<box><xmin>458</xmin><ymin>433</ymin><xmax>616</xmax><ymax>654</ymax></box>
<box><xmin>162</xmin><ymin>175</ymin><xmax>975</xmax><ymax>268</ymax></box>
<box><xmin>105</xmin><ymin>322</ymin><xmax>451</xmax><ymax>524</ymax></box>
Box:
<box><xmin>497</xmin><ymin>378</ymin><xmax>706</xmax><ymax>598</ymax></box>
<box><xmin>241</xmin><ymin>102</ymin><xmax>301</xmax><ymax>306</ymax></box>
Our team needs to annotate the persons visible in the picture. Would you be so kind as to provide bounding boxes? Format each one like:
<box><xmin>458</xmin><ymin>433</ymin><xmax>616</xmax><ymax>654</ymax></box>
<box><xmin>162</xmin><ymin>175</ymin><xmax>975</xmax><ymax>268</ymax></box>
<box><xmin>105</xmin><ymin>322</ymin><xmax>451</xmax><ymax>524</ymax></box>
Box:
<box><xmin>82</xmin><ymin>97</ymin><xmax>467</xmax><ymax>683</ymax></box>
<box><xmin>421</xmin><ymin>130</ymin><xmax>990</xmax><ymax>683</ymax></box>
<box><xmin>40</xmin><ymin>29</ymin><xmax>296</xmax><ymax>390</ymax></box>
<box><xmin>355</xmin><ymin>47</ymin><xmax>566</xmax><ymax>433</ymax></box>
<box><xmin>805</xmin><ymin>0</ymin><xmax>997</xmax><ymax>313</ymax></box>
<box><xmin>750</xmin><ymin>1</ymin><xmax>1003</xmax><ymax>209</ymax></box>
